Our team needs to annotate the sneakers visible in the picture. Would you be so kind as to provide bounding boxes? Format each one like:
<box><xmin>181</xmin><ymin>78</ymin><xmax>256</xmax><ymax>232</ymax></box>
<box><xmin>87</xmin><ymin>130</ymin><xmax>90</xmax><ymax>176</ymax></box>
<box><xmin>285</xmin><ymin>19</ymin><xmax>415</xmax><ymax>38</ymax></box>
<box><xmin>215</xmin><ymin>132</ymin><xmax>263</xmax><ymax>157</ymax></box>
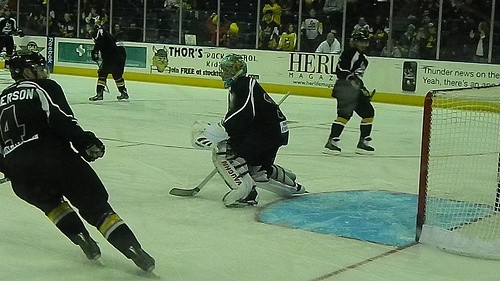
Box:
<box><xmin>124</xmin><ymin>243</ymin><xmax>159</xmax><ymax>278</ymax></box>
<box><xmin>322</xmin><ymin>135</ymin><xmax>341</xmax><ymax>155</ymax></box>
<box><xmin>76</xmin><ymin>232</ymin><xmax>105</xmax><ymax>266</ymax></box>
<box><xmin>292</xmin><ymin>182</ymin><xmax>309</xmax><ymax>194</ymax></box>
<box><xmin>89</xmin><ymin>90</ymin><xmax>103</xmax><ymax>104</ymax></box>
<box><xmin>117</xmin><ymin>88</ymin><xmax>129</xmax><ymax>102</ymax></box>
<box><xmin>355</xmin><ymin>136</ymin><xmax>374</xmax><ymax>156</ymax></box>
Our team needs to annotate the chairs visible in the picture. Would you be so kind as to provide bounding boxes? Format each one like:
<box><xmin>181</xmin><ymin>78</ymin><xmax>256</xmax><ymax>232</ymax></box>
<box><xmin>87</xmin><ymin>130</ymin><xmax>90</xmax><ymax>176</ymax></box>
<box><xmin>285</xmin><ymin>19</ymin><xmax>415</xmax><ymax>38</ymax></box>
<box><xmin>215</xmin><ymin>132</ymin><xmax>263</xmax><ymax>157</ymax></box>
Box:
<box><xmin>393</xmin><ymin>0</ymin><xmax>500</xmax><ymax>63</ymax></box>
<box><xmin>115</xmin><ymin>0</ymin><xmax>256</xmax><ymax>48</ymax></box>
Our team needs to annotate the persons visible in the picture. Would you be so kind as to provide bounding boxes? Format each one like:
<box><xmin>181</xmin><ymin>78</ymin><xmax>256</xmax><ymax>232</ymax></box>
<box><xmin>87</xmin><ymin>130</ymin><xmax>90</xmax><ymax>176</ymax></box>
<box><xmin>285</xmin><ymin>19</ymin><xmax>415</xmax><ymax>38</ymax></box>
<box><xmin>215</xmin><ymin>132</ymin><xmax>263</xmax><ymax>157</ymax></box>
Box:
<box><xmin>190</xmin><ymin>53</ymin><xmax>306</xmax><ymax>207</ymax></box>
<box><xmin>0</xmin><ymin>50</ymin><xmax>157</xmax><ymax>280</ymax></box>
<box><xmin>324</xmin><ymin>29</ymin><xmax>376</xmax><ymax>155</ymax></box>
<box><xmin>88</xmin><ymin>24</ymin><xmax>130</xmax><ymax>104</ymax></box>
<box><xmin>1</xmin><ymin>0</ymin><xmax>500</xmax><ymax>64</ymax></box>
<box><xmin>0</xmin><ymin>9</ymin><xmax>24</xmax><ymax>70</ymax></box>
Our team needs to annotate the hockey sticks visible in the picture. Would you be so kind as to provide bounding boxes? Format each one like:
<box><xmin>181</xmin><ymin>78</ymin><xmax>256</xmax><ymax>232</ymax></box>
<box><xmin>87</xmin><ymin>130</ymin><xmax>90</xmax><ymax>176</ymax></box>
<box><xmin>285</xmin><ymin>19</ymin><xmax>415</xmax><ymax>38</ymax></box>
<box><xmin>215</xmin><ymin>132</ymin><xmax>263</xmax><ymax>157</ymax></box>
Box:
<box><xmin>169</xmin><ymin>91</ymin><xmax>289</xmax><ymax>196</ymax></box>
<box><xmin>0</xmin><ymin>151</ymin><xmax>86</xmax><ymax>184</ymax></box>
<box><xmin>96</xmin><ymin>61</ymin><xmax>109</xmax><ymax>93</ymax></box>
<box><xmin>370</xmin><ymin>89</ymin><xmax>376</xmax><ymax>98</ymax></box>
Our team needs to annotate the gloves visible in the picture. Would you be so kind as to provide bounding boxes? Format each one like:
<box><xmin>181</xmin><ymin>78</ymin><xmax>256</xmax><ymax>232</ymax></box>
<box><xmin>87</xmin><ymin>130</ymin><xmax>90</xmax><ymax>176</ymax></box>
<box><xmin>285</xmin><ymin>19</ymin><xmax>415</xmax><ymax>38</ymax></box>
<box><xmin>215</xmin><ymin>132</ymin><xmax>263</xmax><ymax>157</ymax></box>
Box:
<box><xmin>91</xmin><ymin>50</ymin><xmax>99</xmax><ymax>62</ymax></box>
<box><xmin>72</xmin><ymin>130</ymin><xmax>105</xmax><ymax>162</ymax></box>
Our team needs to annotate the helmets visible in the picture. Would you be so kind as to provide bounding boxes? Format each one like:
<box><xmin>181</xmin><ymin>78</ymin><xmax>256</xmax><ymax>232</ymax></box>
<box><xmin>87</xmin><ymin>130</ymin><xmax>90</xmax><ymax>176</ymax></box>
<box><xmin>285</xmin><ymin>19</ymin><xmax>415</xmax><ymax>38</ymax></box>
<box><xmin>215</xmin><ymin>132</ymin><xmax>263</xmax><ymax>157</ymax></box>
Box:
<box><xmin>5</xmin><ymin>7</ymin><xmax>11</xmax><ymax>11</ymax></box>
<box><xmin>350</xmin><ymin>28</ymin><xmax>370</xmax><ymax>40</ymax></box>
<box><xmin>219</xmin><ymin>53</ymin><xmax>247</xmax><ymax>88</ymax></box>
<box><xmin>9</xmin><ymin>50</ymin><xmax>46</xmax><ymax>69</ymax></box>
<box><xmin>90</xmin><ymin>24</ymin><xmax>102</xmax><ymax>38</ymax></box>
